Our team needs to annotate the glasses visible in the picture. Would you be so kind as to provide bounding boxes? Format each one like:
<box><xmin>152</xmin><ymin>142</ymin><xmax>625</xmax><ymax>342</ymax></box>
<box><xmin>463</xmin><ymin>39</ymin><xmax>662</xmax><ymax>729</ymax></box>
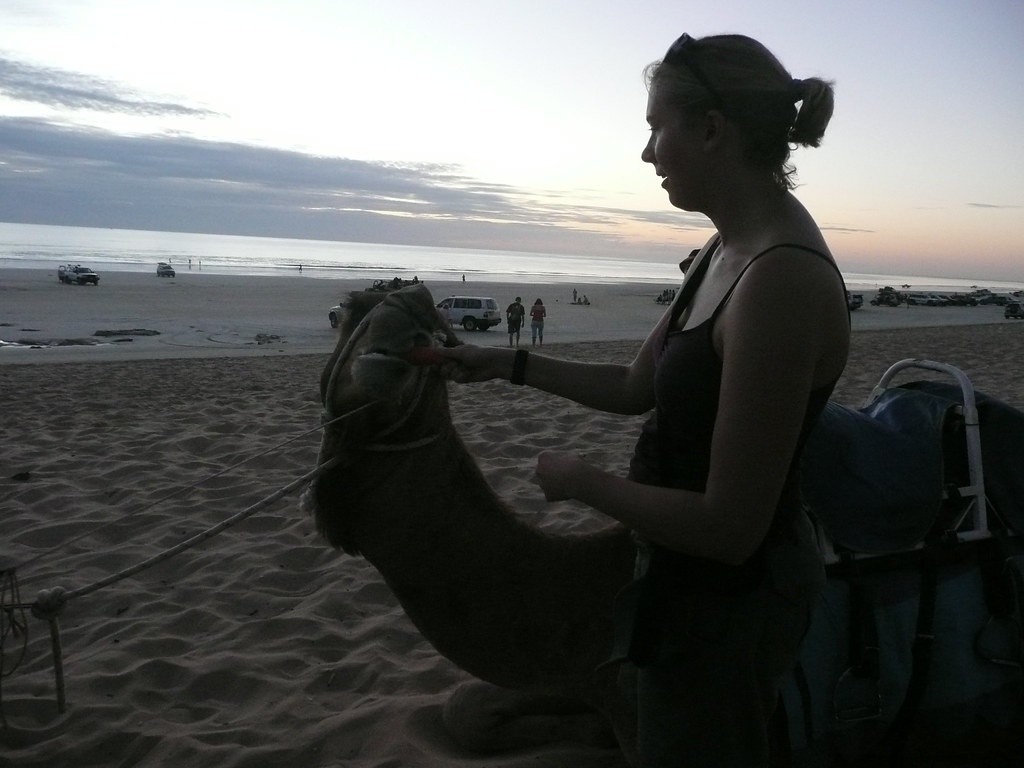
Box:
<box><xmin>663</xmin><ymin>33</ymin><xmax>736</xmax><ymax>120</ymax></box>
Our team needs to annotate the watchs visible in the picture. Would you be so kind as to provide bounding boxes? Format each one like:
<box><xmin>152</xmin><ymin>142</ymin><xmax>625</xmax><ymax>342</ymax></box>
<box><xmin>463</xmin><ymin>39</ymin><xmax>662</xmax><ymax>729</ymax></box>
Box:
<box><xmin>504</xmin><ymin>348</ymin><xmax>530</xmax><ymax>389</ymax></box>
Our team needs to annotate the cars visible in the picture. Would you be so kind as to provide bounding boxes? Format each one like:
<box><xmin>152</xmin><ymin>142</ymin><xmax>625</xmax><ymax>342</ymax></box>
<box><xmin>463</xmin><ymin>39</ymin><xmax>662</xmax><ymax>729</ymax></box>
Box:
<box><xmin>328</xmin><ymin>303</ymin><xmax>343</xmax><ymax>328</ymax></box>
<box><xmin>844</xmin><ymin>286</ymin><xmax>1019</xmax><ymax>308</ymax></box>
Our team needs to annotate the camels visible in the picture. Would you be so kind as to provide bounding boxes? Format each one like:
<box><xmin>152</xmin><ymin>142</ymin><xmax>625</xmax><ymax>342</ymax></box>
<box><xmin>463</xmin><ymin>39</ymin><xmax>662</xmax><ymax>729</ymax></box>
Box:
<box><xmin>304</xmin><ymin>275</ymin><xmax>1023</xmax><ymax>767</ymax></box>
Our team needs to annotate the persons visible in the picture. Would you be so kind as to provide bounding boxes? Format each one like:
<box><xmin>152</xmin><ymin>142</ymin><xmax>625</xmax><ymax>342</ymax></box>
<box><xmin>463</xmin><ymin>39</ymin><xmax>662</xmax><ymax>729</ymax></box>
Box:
<box><xmin>299</xmin><ymin>264</ymin><xmax>302</xmax><ymax>274</ymax></box>
<box><xmin>530</xmin><ymin>298</ymin><xmax>546</xmax><ymax>347</ymax></box>
<box><xmin>572</xmin><ymin>288</ymin><xmax>590</xmax><ymax>305</ymax></box>
<box><xmin>906</xmin><ymin>294</ymin><xmax>911</xmax><ymax>308</ymax></box>
<box><xmin>505</xmin><ymin>296</ymin><xmax>525</xmax><ymax>346</ymax></box>
<box><xmin>657</xmin><ymin>288</ymin><xmax>676</xmax><ymax>304</ymax></box>
<box><xmin>433</xmin><ymin>34</ymin><xmax>854</xmax><ymax>768</ymax></box>
<box><xmin>462</xmin><ymin>274</ymin><xmax>465</xmax><ymax>283</ymax></box>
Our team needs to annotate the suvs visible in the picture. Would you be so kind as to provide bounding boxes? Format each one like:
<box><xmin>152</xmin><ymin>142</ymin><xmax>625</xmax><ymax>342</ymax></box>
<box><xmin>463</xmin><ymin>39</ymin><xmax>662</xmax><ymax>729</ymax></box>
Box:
<box><xmin>156</xmin><ymin>262</ymin><xmax>175</xmax><ymax>278</ymax></box>
<box><xmin>1004</xmin><ymin>300</ymin><xmax>1024</xmax><ymax>318</ymax></box>
<box><xmin>434</xmin><ymin>295</ymin><xmax>502</xmax><ymax>332</ymax></box>
<box><xmin>58</xmin><ymin>265</ymin><xmax>100</xmax><ymax>286</ymax></box>
